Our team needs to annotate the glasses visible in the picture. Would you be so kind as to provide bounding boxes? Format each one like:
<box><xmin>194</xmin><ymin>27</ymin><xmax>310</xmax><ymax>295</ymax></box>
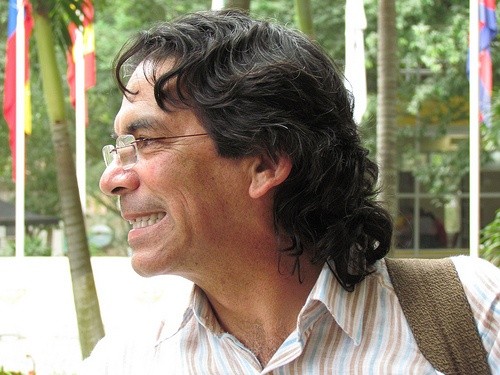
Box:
<box><xmin>101</xmin><ymin>121</ymin><xmax>263</xmax><ymax>171</ymax></box>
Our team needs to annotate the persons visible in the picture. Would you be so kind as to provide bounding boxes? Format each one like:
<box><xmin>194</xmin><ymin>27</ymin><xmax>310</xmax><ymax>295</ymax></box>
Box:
<box><xmin>58</xmin><ymin>8</ymin><xmax>500</xmax><ymax>374</ymax></box>
<box><xmin>396</xmin><ymin>207</ymin><xmax>445</xmax><ymax>249</ymax></box>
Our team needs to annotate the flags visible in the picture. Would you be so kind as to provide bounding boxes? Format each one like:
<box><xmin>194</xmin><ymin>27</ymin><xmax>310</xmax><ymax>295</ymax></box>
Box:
<box><xmin>342</xmin><ymin>2</ymin><xmax>370</xmax><ymax>124</ymax></box>
<box><xmin>3</xmin><ymin>0</ymin><xmax>36</xmax><ymax>183</ymax></box>
<box><xmin>57</xmin><ymin>0</ymin><xmax>98</xmax><ymax>126</ymax></box>
<box><xmin>467</xmin><ymin>0</ymin><xmax>499</xmax><ymax>127</ymax></box>
